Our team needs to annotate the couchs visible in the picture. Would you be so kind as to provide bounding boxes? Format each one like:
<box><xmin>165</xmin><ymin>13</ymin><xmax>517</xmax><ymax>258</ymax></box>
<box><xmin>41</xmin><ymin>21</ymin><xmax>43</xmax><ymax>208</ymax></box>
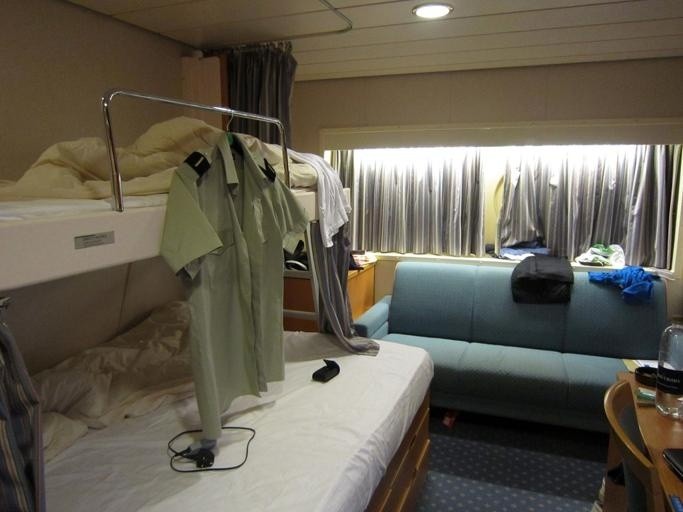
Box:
<box><xmin>351</xmin><ymin>260</ymin><xmax>667</xmax><ymax>434</ymax></box>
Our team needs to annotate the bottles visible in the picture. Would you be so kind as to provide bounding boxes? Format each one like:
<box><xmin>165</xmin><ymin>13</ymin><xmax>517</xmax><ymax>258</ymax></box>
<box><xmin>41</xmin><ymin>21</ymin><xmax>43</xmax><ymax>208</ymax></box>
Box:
<box><xmin>656</xmin><ymin>317</ymin><xmax>683</xmax><ymax>416</ymax></box>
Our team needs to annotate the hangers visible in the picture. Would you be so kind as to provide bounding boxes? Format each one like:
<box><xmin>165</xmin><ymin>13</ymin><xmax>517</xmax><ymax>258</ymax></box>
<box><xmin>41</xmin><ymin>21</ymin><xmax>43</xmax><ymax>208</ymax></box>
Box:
<box><xmin>221</xmin><ymin>104</ymin><xmax>245</xmax><ymax>158</ymax></box>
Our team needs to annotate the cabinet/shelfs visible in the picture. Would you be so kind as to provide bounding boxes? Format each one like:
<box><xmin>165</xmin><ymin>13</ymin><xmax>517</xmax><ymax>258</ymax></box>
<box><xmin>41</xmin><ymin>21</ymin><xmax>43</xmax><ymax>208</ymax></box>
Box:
<box><xmin>283</xmin><ymin>263</ymin><xmax>375</xmax><ymax>332</ymax></box>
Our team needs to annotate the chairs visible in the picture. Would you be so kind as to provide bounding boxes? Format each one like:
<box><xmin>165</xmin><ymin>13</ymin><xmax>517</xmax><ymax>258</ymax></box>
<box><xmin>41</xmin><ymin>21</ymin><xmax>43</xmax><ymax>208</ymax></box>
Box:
<box><xmin>598</xmin><ymin>380</ymin><xmax>665</xmax><ymax>512</ymax></box>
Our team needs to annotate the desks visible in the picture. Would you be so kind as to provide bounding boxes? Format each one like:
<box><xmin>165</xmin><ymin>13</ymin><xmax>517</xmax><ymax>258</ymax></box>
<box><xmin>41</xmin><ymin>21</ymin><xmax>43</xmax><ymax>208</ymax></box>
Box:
<box><xmin>617</xmin><ymin>371</ymin><xmax>683</xmax><ymax>512</ymax></box>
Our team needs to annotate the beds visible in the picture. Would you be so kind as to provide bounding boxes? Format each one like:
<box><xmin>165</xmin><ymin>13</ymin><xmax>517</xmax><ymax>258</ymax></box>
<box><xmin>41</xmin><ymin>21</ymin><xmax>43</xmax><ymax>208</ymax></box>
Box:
<box><xmin>0</xmin><ymin>88</ymin><xmax>455</xmax><ymax>512</ymax></box>
